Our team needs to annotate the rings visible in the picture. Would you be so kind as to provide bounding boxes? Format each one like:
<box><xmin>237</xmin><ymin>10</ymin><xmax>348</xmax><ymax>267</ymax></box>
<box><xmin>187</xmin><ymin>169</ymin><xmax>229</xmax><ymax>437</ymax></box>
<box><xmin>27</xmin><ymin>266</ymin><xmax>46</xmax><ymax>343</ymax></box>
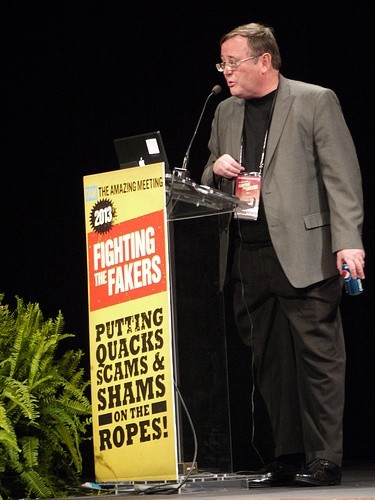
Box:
<box><xmin>361</xmin><ymin>261</ymin><xmax>364</xmax><ymax>264</ymax></box>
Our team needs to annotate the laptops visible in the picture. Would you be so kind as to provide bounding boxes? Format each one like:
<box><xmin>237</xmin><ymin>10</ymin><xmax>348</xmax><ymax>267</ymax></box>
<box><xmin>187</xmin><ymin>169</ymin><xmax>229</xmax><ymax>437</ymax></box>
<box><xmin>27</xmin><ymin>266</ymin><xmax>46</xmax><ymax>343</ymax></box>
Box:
<box><xmin>114</xmin><ymin>131</ymin><xmax>171</xmax><ymax>175</ymax></box>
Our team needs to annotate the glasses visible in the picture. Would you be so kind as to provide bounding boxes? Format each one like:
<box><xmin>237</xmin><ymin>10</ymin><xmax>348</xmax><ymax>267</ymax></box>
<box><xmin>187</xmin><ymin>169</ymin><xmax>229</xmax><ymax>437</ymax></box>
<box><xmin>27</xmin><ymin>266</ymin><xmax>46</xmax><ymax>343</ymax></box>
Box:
<box><xmin>215</xmin><ymin>54</ymin><xmax>253</xmax><ymax>73</ymax></box>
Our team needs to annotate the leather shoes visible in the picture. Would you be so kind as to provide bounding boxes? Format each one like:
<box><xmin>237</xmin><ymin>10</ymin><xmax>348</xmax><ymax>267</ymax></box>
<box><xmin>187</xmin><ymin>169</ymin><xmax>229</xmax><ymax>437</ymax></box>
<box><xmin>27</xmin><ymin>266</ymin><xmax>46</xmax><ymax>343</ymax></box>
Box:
<box><xmin>293</xmin><ymin>462</ymin><xmax>344</xmax><ymax>486</ymax></box>
<box><xmin>242</xmin><ymin>464</ymin><xmax>293</xmax><ymax>487</ymax></box>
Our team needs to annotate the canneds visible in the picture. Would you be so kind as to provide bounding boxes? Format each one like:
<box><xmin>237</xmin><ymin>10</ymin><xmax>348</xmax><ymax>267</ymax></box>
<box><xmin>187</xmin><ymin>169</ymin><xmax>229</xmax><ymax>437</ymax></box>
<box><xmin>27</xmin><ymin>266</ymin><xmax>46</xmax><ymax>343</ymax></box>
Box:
<box><xmin>342</xmin><ymin>264</ymin><xmax>363</xmax><ymax>295</ymax></box>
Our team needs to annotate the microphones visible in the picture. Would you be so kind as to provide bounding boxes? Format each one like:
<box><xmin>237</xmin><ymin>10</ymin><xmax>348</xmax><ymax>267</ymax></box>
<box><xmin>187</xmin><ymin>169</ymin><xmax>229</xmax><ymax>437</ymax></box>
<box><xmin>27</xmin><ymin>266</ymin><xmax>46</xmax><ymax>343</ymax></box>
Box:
<box><xmin>173</xmin><ymin>85</ymin><xmax>222</xmax><ymax>178</ymax></box>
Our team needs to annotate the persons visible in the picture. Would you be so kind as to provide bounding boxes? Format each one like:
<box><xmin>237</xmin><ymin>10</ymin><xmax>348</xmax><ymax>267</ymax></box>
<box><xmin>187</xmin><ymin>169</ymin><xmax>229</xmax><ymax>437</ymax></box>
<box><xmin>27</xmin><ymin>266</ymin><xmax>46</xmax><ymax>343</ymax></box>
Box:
<box><xmin>200</xmin><ymin>23</ymin><xmax>367</xmax><ymax>488</ymax></box>
